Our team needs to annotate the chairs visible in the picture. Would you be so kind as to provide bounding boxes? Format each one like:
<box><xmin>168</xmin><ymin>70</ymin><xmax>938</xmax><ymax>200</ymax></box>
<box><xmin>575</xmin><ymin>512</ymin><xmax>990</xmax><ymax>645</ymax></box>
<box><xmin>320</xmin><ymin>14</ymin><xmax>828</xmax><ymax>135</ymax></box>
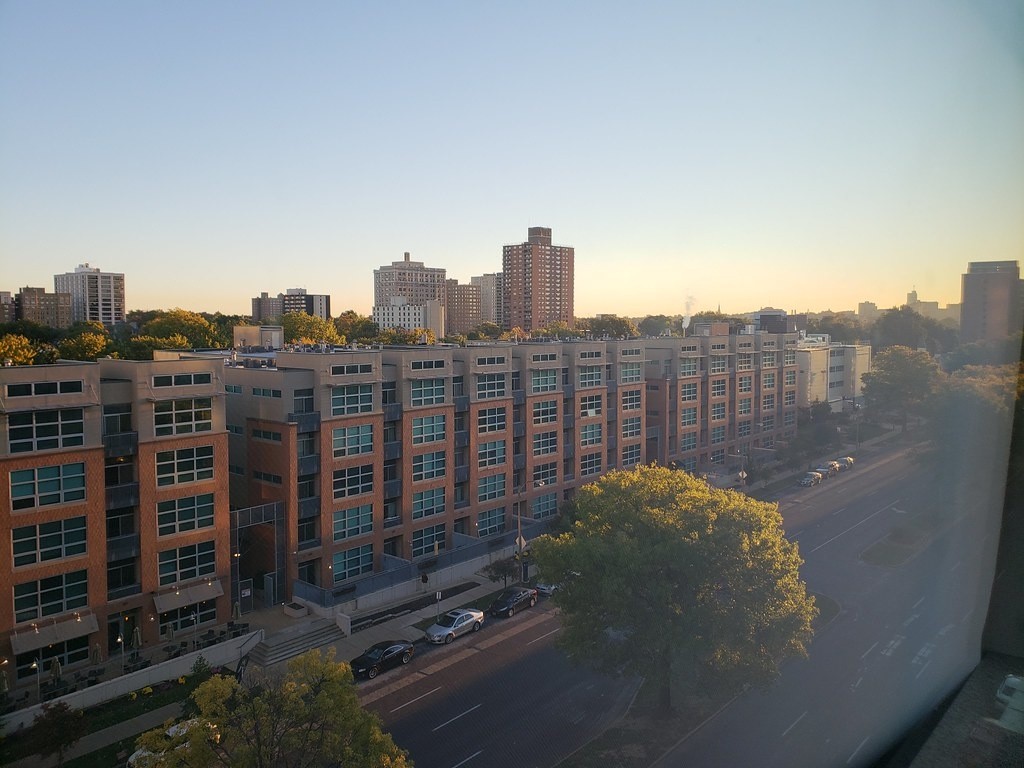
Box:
<box><xmin>0</xmin><ymin>620</ymin><xmax>251</xmax><ymax>713</ymax></box>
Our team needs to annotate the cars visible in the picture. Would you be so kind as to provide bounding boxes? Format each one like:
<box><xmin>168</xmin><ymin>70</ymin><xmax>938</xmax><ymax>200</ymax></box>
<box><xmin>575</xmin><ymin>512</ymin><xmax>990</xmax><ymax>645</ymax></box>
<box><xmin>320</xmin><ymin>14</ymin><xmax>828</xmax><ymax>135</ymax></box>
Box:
<box><xmin>536</xmin><ymin>582</ymin><xmax>562</xmax><ymax>596</ymax></box>
<box><xmin>489</xmin><ymin>586</ymin><xmax>538</xmax><ymax>618</ymax></box>
<box><xmin>816</xmin><ymin>461</ymin><xmax>840</xmax><ymax>479</ymax></box>
<box><xmin>125</xmin><ymin>718</ymin><xmax>220</xmax><ymax>768</ymax></box>
<box><xmin>349</xmin><ymin>639</ymin><xmax>414</xmax><ymax>679</ymax></box>
<box><xmin>802</xmin><ymin>470</ymin><xmax>822</xmax><ymax>487</ymax></box>
<box><xmin>425</xmin><ymin>608</ymin><xmax>486</xmax><ymax>644</ymax></box>
<box><xmin>836</xmin><ymin>457</ymin><xmax>854</xmax><ymax>472</ymax></box>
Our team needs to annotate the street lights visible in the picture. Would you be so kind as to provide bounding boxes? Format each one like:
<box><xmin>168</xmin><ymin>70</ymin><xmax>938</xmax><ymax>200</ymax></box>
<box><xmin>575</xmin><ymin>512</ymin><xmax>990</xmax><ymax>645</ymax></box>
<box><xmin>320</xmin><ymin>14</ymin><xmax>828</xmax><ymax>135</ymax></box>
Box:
<box><xmin>190</xmin><ymin>610</ymin><xmax>196</xmax><ymax>651</ymax></box>
<box><xmin>30</xmin><ymin>657</ymin><xmax>40</xmax><ymax>703</ymax></box>
<box><xmin>741</xmin><ymin>422</ymin><xmax>763</xmax><ymax>492</ymax></box>
<box><xmin>517</xmin><ymin>480</ymin><xmax>546</xmax><ymax>563</ymax></box>
<box><xmin>117</xmin><ymin>633</ymin><xmax>124</xmax><ymax>676</ymax></box>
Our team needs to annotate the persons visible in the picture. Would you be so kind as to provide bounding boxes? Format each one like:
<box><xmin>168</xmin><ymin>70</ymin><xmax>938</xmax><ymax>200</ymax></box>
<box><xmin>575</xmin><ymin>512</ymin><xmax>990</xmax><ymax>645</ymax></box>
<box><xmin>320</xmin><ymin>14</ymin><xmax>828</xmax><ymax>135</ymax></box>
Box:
<box><xmin>422</xmin><ymin>572</ymin><xmax>429</xmax><ymax>594</ymax></box>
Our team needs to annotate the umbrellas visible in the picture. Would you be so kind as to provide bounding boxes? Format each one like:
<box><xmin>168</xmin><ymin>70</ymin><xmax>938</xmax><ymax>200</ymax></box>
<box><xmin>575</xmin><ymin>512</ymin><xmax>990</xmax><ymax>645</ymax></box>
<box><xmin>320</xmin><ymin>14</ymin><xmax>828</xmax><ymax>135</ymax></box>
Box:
<box><xmin>131</xmin><ymin>627</ymin><xmax>143</xmax><ymax>659</ymax></box>
<box><xmin>164</xmin><ymin>622</ymin><xmax>175</xmax><ymax>642</ymax></box>
<box><xmin>91</xmin><ymin>644</ymin><xmax>103</xmax><ymax>672</ymax></box>
<box><xmin>232</xmin><ymin>602</ymin><xmax>242</xmax><ymax>620</ymax></box>
<box><xmin>0</xmin><ymin>671</ymin><xmax>9</xmax><ymax>695</ymax></box>
<box><xmin>49</xmin><ymin>656</ymin><xmax>62</xmax><ymax>685</ymax></box>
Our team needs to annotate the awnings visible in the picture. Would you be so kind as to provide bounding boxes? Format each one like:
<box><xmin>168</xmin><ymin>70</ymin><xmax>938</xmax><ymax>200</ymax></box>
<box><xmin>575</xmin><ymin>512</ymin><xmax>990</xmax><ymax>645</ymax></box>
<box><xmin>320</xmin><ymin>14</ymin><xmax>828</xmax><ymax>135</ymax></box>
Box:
<box><xmin>153</xmin><ymin>580</ymin><xmax>224</xmax><ymax>614</ymax></box>
<box><xmin>10</xmin><ymin>614</ymin><xmax>99</xmax><ymax>655</ymax></box>
